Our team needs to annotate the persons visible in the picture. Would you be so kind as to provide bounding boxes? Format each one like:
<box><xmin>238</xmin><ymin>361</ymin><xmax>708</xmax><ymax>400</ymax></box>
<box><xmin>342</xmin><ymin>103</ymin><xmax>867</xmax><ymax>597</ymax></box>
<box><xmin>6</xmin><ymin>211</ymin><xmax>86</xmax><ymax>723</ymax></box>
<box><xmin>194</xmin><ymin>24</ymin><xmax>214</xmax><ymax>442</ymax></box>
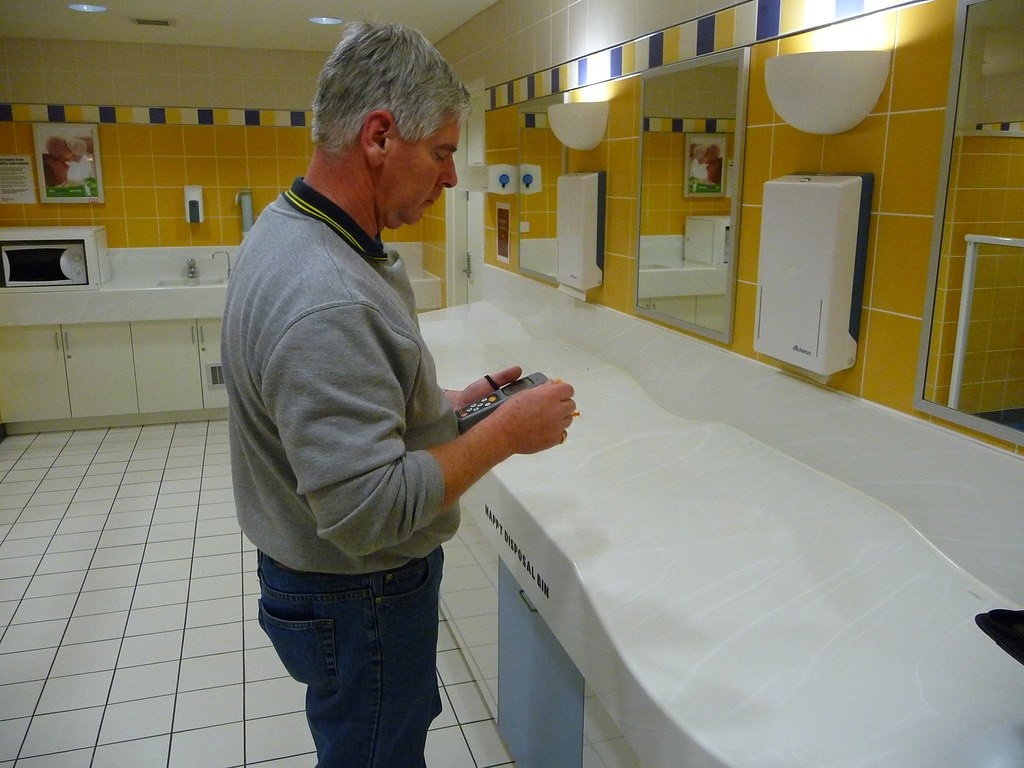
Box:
<box><xmin>221</xmin><ymin>23</ymin><xmax>576</xmax><ymax>768</ymax></box>
<box><xmin>41</xmin><ymin>136</ymin><xmax>93</xmax><ymax>186</ymax></box>
<box><xmin>689</xmin><ymin>143</ymin><xmax>722</xmax><ymax>185</ymax></box>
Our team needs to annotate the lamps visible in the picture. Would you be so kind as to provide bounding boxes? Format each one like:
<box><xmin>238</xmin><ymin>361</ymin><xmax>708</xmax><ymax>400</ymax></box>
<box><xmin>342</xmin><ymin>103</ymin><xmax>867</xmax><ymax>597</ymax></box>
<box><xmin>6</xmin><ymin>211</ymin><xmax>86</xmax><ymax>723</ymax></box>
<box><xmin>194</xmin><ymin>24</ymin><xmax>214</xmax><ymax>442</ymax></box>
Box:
<box><xmin>547</xmin><ymin>101</ymin><xmax>610</xmax><ymax>152</ymax></box>
<box><xmin>763</xmin><ymin>51</ymin><xmax>890</xmax><ymax>135</ymax></box>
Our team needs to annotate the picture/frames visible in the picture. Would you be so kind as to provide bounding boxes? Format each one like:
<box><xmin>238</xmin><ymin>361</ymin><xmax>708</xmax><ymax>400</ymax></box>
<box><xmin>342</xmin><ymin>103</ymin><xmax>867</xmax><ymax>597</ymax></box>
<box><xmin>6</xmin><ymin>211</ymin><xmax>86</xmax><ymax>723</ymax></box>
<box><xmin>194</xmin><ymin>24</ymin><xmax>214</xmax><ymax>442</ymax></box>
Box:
<box><xmin>683</xmin><ymin>133</ymin><xmax>727</xmax><ymax>198</ymax></box>
<box><xmin>32</xmin><ymin>122</ymin><xmax>104</xmax><ymax>204</ymax></box>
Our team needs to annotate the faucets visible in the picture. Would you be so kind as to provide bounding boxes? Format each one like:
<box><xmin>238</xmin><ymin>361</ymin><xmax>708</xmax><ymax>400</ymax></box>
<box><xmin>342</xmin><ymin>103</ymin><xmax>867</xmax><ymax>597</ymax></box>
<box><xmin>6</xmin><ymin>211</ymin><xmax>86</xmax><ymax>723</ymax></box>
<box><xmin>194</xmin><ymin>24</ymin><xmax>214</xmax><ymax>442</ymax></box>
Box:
<box><xmin>212</xmin><ymin>251</ymin><xmax>233</xmax><ymax>279</ymax></box>
<box><xmin>187</xmin><ymin>258</ymin><xmax>196</xmax><ymax>277</ymax></box>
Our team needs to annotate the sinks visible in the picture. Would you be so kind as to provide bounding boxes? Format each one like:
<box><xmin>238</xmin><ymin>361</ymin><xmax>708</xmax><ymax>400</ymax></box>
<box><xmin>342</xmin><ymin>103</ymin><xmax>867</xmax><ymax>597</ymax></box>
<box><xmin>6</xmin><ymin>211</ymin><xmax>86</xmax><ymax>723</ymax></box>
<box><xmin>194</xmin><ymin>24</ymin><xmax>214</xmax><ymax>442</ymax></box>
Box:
<box><xmin>155</xmin><ymin>279</ymin><xmax>224</xmax><ymax>286</ymax></box>
<box><xmin>407</xmin><ymin>271</ymin><xmax>437</xmax><ymax>279</ymax></box>
<box><xmin>638</xmin><ymin>263</ymin><xmax>674</xmax><ymax>271</ymax></box>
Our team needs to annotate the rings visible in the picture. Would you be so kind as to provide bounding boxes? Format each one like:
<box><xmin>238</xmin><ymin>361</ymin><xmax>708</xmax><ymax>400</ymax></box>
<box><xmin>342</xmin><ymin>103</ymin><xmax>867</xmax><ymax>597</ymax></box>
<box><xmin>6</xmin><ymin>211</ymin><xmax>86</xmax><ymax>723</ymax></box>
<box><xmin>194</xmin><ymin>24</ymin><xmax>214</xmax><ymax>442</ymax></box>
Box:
<box><xmin>484</xmin><ymin>375</ymin><xmax>499</xmax><ymax>391</ymax></box>
<box><xmin>561</xmin><ymin>433</ymin><xmax>565</xmax><ymax>444</ymax></box>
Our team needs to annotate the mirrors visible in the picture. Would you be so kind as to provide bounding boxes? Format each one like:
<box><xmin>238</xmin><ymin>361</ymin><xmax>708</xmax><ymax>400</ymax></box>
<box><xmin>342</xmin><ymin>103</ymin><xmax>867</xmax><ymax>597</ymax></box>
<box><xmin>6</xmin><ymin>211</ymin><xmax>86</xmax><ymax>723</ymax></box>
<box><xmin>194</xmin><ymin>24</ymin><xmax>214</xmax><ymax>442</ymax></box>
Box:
<box><xmin>914</xmin><ymin>1</ymin><xmax>1023</xmax><ymax>444</ymax></box>
<box><xmin>633</xmin><ymin>47</ymin><xmax>751</xmax><ymax>344</ymax></box>
<box><xmin>519</xmin><ymin>91</ymin><xmax>568</xmax><ymax>283</ymax></box>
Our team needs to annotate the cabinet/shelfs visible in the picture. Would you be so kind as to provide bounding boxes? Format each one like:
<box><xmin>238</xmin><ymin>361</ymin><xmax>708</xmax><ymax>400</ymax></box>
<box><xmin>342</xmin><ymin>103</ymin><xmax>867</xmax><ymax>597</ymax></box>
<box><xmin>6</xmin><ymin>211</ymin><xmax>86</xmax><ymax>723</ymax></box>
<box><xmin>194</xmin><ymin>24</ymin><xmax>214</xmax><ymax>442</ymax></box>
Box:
<box><xmin>496</xmin><ymin>555</ymin><xmax>585</xmax><ymax>768</ymax></box>
<box><xmin>0</xmin><ymin>319</ymin><xmax>231</xmax><ymax>424</ymax></box>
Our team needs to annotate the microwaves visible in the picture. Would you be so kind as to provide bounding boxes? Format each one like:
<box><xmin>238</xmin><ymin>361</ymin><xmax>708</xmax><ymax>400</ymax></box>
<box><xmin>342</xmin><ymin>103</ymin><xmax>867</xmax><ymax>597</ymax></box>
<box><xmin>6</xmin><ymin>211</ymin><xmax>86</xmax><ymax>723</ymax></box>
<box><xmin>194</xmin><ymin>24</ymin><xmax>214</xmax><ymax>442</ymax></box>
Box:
<box><xmin>0</xmin><ymin>224</ymin><xmax>112</xmax><ymax>293</ymax></box>
